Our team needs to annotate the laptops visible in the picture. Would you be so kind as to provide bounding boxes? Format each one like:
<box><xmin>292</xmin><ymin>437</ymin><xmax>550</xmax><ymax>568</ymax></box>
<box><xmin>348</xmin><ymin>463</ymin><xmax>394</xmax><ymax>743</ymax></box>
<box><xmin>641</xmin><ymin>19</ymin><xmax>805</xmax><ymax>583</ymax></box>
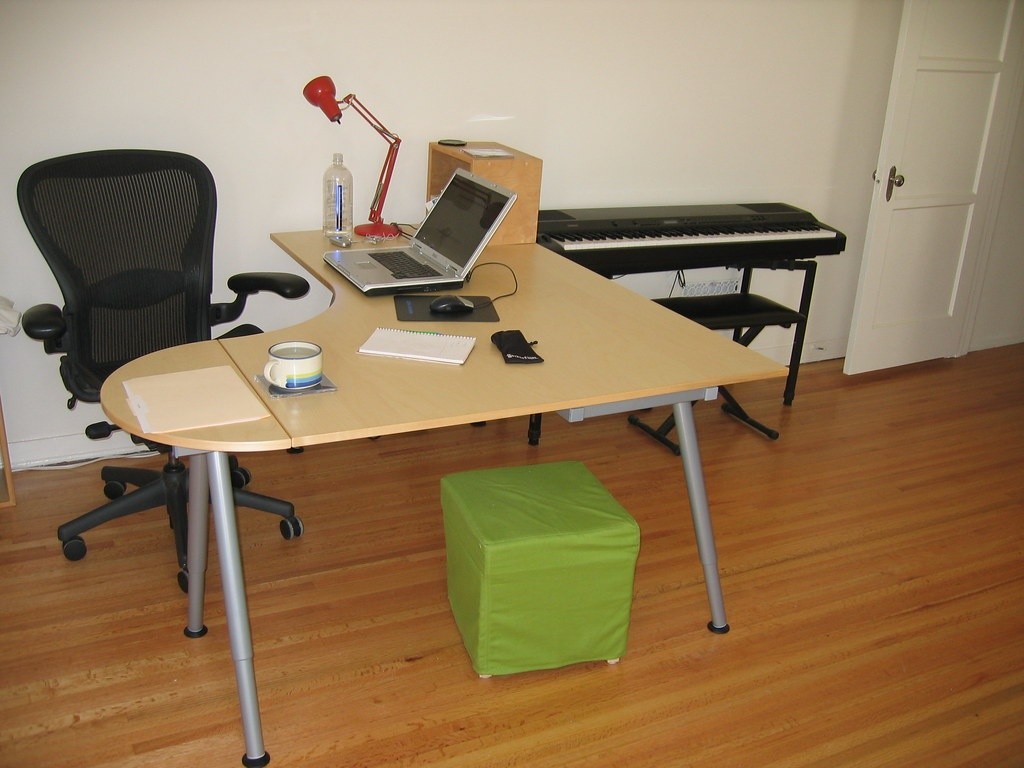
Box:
<box><xmin>323</xmin><ymin>168</ymin><xmax>518</xmax><ymax>296</ymax></box>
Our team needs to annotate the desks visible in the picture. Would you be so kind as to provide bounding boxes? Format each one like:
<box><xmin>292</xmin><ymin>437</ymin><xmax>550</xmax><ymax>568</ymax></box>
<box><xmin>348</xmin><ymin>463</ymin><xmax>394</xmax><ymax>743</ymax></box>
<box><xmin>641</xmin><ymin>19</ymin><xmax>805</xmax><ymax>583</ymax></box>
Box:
<box><xmin>100</xmin><ymin>223</ymin><xmax>789</xmax><ymax>768</ymax></box>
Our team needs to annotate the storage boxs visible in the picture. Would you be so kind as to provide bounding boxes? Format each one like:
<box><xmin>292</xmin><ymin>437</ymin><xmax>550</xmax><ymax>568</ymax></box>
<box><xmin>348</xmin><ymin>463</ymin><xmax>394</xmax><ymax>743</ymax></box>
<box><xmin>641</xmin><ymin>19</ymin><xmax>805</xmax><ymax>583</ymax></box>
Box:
<box><xmin>426</xmin><ymin>141</ymin><xmax>542</xmax><ymax>247</ymax></box>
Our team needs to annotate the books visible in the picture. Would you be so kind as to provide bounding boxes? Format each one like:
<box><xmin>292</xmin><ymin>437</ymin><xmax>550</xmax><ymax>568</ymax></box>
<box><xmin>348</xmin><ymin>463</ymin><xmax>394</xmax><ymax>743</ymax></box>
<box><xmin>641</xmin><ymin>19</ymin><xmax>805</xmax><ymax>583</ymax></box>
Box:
<box><xmin>355</xmin><ymin>326</ymin><xmax>477</xmax><ymax>365</ymax></box>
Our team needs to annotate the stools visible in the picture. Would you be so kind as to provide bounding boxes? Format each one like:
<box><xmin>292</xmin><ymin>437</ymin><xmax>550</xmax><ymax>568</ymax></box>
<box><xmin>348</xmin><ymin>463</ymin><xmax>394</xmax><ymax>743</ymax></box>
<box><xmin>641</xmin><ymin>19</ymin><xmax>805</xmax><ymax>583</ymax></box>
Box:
<box><xmin>440</xmin><ymin>461</ymin><xmax>638</xmax><ymax>680</ymax></box>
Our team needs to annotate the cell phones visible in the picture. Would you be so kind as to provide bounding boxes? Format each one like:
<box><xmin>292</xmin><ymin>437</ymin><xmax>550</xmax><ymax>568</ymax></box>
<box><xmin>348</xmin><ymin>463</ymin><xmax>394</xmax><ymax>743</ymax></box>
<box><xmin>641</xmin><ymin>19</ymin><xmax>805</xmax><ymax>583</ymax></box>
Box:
<box><xmin>329</xmin><ymin>236</ymin><xmax>353</xmax><ymax>248</ymax></box>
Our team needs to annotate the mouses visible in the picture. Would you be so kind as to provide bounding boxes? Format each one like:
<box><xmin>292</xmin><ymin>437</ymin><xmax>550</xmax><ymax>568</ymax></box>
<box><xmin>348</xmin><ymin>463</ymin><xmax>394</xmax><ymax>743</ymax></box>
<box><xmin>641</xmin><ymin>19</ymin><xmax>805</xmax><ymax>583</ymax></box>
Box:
<box><xmin>429</xmin><ymin>295</ymin><xmax>474</xmax><ymax>313</ymax></box>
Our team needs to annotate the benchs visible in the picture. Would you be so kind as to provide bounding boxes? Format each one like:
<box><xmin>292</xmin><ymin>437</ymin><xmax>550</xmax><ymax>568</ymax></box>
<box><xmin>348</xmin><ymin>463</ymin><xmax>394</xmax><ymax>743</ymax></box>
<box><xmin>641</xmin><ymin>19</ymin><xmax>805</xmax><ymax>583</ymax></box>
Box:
<box><xmin>629</xmin><ymin>294</ymin><xmax>805</xmax><ymax>456</ymax></box>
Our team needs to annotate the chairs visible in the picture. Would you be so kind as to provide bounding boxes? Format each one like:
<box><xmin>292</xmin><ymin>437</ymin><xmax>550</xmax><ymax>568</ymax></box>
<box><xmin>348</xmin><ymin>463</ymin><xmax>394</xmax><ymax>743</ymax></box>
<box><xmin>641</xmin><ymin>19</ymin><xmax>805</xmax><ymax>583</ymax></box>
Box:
<box><xmin>18</xmin><ymin>148</ymin><xmax>303</xmax><ymax>594</ymax></box>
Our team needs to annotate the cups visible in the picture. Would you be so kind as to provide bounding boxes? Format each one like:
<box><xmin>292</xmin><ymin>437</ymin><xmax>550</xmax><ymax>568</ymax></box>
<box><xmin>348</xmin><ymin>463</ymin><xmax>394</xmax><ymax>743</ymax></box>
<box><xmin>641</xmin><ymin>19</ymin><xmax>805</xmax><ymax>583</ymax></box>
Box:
<box><xmin>264</xmin><ymin>341</ymin><xmax>322</xmax><ymax>389</ymax></box>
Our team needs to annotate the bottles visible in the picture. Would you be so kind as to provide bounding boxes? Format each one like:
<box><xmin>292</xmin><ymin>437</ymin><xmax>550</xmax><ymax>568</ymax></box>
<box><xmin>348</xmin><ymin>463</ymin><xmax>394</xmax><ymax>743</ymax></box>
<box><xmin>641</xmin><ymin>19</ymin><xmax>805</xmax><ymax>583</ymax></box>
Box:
<box><xmin>322</xmin><ymin>154</ymin><xmax>353</xmax><ymax>239</ymax></box>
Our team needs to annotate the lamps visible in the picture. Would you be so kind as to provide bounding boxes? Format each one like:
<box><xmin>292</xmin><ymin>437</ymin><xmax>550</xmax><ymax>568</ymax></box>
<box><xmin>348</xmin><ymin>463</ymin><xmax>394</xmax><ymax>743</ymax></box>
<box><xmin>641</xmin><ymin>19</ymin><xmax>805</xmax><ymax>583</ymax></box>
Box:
<box><xmin>303</xmin><ymin>76</ymin><xmax>401</xmax><ymax>240</ymax></box>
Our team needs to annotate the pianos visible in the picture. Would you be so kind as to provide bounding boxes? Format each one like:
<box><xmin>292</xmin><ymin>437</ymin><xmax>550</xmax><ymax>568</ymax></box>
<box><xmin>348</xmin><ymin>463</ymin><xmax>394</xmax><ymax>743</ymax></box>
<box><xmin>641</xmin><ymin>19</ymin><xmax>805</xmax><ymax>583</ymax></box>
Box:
<box><xmin>535</xmin><ymin>203</ymin><xmax>847</xmax><ymax>275</ymax></box>
